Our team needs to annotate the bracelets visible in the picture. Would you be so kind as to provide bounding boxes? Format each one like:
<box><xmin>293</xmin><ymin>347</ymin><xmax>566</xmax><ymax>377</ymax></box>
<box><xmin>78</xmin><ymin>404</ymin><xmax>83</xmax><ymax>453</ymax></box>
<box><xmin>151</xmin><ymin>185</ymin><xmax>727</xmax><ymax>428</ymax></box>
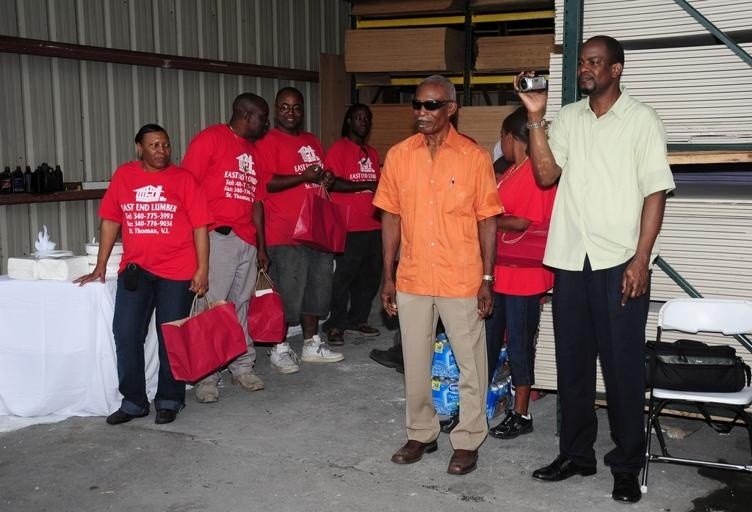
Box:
<box><xmin>525</xmin><ymin>119</ymin><xmax>547</xmax><ymax>131</ymax></box>
<box><xmin>483</xmin><ymin>274</ymin><xmax>496</xmax><ymax>282</ymax></box>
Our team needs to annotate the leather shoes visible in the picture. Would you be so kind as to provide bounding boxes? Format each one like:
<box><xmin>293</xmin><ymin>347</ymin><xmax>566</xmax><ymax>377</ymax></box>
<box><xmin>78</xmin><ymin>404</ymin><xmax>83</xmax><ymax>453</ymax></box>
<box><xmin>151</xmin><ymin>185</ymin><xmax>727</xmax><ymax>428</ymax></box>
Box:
<box><xmin>447</xmin><ymin>449</ymin><xmax>478</xmax><ymax>475</ymax></box>
<box><xmin>369</xmin><ymin>344</ymin><xmax>403</xmax><ymax>373</ymax></box>
<box><xmin>532</xmin><ymin>456</ymin><xmax>597</xmax><ymax>481</ymax></box>
<box><xmin>612</xmin><ymin>473</ymin><xmax>642</xmax><ymax>503</ymax></box>
<box><xmin>106</xmin><ymin>409</ymin><xmax>131</xmax><ymax>424</ymax></box>
<box><xmin>345</xmin><ymin>325</ymin><xmax>380</xmax><ymax>336</ymax></box>
<box><xmin>328</xmin><ymin>329</ymin><xmax>344</xmax><ymax>344</ymax></box>
<box><xmin>154</xmin><ymin>409</ymin><xmax>176</xmax><ymax>424</ymax></box>
<box><xmin>391</xmin><ymin>439</ymin><xmax>438</xmax><ymax>464</ymax></box>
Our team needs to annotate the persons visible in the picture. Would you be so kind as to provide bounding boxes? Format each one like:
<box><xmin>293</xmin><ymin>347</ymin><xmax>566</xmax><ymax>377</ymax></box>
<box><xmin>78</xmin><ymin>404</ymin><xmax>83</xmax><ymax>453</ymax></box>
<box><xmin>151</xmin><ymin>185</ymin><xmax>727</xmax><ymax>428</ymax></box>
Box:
<box><xmin>325</xmin><ymin>102</ymin><xmax>383</xmax><ymax>345</ymax></box>
<box><xmin>510</xmin><ymin>33</ymin><xmax>675</xmax><ymax>507</ymax></box>
<box><xmin>439</xmin><ymin>103</ymin><xmax>559</xmax><ymax>439</ymax></box>
<box><xmin>370</xmin><ymin>246</ymin><xmax>446</xmax><ymax>377</ymax></box>
<box><xmin>251</xmin><ymin>87</ymin><xmax>346</xmax><ymax>374</ymax></box>
<box><xmin>369</xmin><ymin>74</ymin><xmax>505</xmax><ymax>474</ymax></box>
<box><xmin>174</xmin><ymin>92</ymin><xmax>270</xmax><ymax>404</ymax></box>
<box><xmin>70</xmin><ymin>121</ymin><xmax>213</xmax><ymax>425</ymax></box>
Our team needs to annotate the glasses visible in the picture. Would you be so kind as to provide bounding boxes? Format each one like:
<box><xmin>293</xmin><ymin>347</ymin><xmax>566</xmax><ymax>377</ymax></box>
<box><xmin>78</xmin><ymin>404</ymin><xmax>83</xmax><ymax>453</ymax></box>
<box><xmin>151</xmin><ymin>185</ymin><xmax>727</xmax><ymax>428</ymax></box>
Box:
<box><xmin>359</xmin><ymin>146</ymin><xmax>368</xmax><ymax>164</ymax></box>
<box><xmin>411</xmin><ymin>98</ymin><xmax>453</xmax><ymax>110</ymax></box>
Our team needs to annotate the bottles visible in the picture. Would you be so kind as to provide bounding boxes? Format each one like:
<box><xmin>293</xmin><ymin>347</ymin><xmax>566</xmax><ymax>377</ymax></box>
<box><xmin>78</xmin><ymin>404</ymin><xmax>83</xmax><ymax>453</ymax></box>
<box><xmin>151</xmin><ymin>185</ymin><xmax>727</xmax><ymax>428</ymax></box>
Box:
<box><xmin>431</xmin><ymin>334</ymin><xmax>516</xmax><ymax>415</ymax></box>
<box><xmin>0</xmin><ymin>162</ymin><xmax>67</xmax><ymax>194</ymax></box>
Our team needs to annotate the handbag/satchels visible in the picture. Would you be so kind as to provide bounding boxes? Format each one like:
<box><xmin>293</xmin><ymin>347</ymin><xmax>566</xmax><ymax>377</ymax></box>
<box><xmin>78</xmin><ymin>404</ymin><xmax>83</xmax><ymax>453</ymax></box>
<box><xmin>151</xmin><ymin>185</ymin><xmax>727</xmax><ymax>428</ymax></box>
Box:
<box><xmin>644</xmin><ymin>339</ymin><xmax>752</xmax><ymax>393</ymax></box>
<box><xmin>247</xmin><ymin>269</ymin><xmax>286</xmax><ymax>343</ymax></box>
<box><xmin>160</xmin><ymin>293</ymin><xmax>248</xmax><ymax>382</ymax></box>
<box><xmin>291</xmin><ymin>180</ymin><xmax>350</xmax><ymax>256</ymax></box>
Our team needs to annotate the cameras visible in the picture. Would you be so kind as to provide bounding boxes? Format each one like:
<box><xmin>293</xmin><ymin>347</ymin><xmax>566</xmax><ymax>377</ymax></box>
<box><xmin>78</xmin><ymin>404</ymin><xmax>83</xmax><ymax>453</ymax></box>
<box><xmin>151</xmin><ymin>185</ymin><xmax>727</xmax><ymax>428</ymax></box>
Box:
<box><xmin>519</xmin><ymin>74</ymin><xmax>548</xmax><ymax>93</ymax></box>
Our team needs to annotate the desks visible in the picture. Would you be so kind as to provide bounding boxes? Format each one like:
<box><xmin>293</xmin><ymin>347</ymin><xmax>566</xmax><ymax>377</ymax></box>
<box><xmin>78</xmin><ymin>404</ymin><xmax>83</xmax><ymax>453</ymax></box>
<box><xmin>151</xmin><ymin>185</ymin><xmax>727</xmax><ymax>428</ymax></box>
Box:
<box><xmin>0</xmin><ymin>268</ymin><xmax>163</xmax><ymax>430</ymax></box>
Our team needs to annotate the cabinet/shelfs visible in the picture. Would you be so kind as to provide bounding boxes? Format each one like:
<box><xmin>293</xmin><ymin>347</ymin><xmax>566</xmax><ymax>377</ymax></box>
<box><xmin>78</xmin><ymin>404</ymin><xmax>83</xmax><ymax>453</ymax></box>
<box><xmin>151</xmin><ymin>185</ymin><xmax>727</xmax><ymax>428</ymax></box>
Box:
<box><xmin>349</xmin><ymin>9</ymin><xmax>556</xmax><ymax>88</ymax></box>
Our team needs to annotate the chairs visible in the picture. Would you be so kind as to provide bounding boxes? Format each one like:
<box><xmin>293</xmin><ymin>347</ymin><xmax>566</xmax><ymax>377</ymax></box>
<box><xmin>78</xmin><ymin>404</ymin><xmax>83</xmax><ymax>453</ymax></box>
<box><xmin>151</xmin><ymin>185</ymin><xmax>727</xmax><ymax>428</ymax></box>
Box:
<box><xmin>638</xmin><ymin>297</ymin><xmax>752</xmax><ymax>492</ymax></box>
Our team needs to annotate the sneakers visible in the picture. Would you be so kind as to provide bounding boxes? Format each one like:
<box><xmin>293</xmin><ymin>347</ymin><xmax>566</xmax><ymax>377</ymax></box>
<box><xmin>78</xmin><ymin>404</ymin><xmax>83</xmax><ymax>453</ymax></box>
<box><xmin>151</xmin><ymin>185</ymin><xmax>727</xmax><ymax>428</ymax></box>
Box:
<box><xmin>195</xmin><ymin>379</ymin><xmax>220</xmax><ymax>403</ymax></box>
<box><xmin>231</xmin><ymin>372</ymin><xmax>264</xmax><ymax>391</ymax></box>
<box><xmin>301</xmin><ymin>335</ymin><xmax>344</xmax><ymax>362</ymax></box>
<box><xmin>439</xmin><ymin>406</ymin><xmax>460</xmax><ymax>433</ymax></box>
<box><xmin>270</xmin><ymin>349</ymin><xmax>301</xmax><ymax>374</ymax></box>
<box><xmin>489</xmin><ymin>409</ymin><xmax>533</xmax><ymax>439</ymax></box>
